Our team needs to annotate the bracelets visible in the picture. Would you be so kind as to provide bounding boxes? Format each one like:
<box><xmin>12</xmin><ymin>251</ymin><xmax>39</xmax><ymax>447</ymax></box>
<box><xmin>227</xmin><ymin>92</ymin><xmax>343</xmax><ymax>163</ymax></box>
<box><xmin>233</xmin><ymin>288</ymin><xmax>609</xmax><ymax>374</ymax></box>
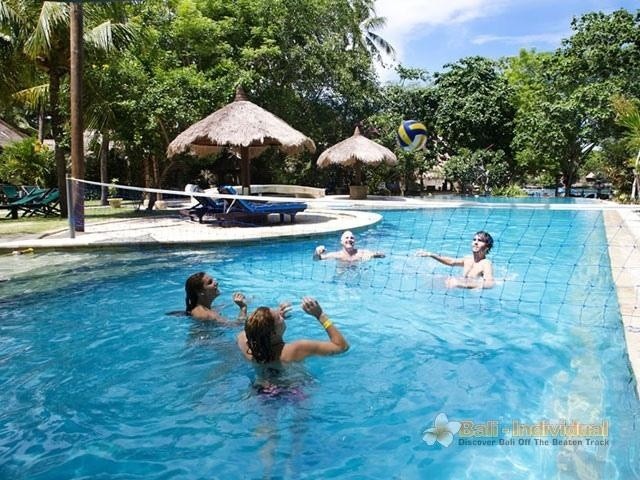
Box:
<box><xmin>322</xmin><ymin>320</ymin><xmax>333</xmax><ymax>329</ymax></box>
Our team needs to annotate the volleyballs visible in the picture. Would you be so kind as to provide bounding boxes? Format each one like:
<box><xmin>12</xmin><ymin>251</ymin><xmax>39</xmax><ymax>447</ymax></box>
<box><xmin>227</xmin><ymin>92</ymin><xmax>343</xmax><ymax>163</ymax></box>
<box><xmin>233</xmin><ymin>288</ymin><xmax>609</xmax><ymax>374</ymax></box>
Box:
<box><xmin>397</xmin><ymin>120</ymin><xmax>428</xmax><ymax>151</ymax></box>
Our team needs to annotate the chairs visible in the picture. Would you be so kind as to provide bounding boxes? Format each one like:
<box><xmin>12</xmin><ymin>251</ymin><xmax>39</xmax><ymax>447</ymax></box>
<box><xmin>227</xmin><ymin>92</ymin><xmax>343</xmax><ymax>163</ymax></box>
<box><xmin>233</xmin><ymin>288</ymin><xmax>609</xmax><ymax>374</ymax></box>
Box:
<box><xmin>187</xmin><ymin>192</ymin><xmax>224</xmax><ymax>223</ymax></box>
<box><xmin>218</xmin><ymin>185</ymin><xmax>307</xmax><ymax>225</ymax></box>
<box><xmin>0</xmin><ymin>183</ymin><xmax>64</xmax><ymax>219</ymax></box>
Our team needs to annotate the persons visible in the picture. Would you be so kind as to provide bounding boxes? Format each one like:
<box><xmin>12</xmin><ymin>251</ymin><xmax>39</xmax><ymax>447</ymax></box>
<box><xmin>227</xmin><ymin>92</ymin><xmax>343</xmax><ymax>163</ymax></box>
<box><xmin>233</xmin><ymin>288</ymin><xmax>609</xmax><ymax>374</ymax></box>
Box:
<box><xmin>238</xmin><ymin>294</ymin><xmax>352</xmax><ymax>480</ymax></box>
<box><xmin>312</xmin><ymin>229</ymin><xmax>387</xmax><ymax>266</ymax></box>
<box><xmin>416</xmin><ymin>231</ymin><xmax>498</xmax><ymax>290</ymax></box>
<box><xmin>168</xmin><ymin>270</ymin><xmax>247</xmax><ymax>329</ymax></box>
